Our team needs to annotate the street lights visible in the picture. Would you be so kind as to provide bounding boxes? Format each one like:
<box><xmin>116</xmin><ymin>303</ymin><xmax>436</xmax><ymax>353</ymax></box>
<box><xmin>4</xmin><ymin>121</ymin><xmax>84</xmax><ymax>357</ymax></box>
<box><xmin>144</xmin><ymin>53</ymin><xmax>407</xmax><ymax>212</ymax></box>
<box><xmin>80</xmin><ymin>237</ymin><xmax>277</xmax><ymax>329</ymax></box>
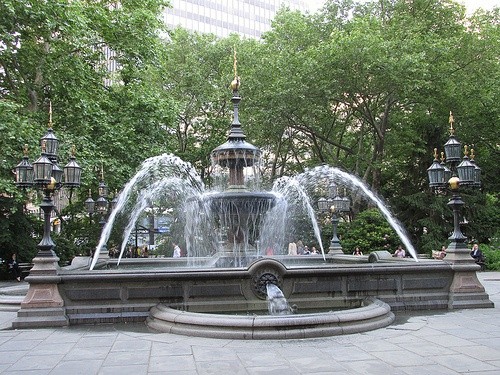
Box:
<box><xmin>317</xmin><ymin>177</ymin><xmax>352</xmax><ymax>255</ymax></box>
<box><xmin>84</xmin><ymin>179</ymin><xmax>119</xmax><ymax>258</ymax></box>
<box><xmin>426</xmin><ymin>110</ymin><xmax>482</xmax><ymax>249</ymax></box>
<box><xmin>13</xmin><ymin>119</ymin><xmax>82</xmax><ymax>257</ymax></box>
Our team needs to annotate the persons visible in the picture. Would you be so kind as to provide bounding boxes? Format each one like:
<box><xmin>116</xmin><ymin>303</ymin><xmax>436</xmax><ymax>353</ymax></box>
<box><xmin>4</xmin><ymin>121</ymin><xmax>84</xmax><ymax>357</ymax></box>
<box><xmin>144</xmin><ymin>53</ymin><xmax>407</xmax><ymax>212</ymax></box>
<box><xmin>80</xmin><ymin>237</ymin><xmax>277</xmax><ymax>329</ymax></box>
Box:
<box><xmin>470</xmin><ymin>244</ymin><xmax>481</xmax><ymax>262</ymax></box>
<box><xmin>439</xmin><ymin>245</ymin><xmax>446</xmax><ymax>258</ymax></box>
<box><xmin>353</xmin><ymin>246</ymin><xmax>362</xmax><ymax>255</ymax></box>
<box><xmin>108</xmin><ymin>244</ymin><xmax>133</xmax><ymax>258</ymax></box>
<box><xmin>172</xmin><ymin>241</ymin><xmax>180</xmax><ymax>257</ymax></box>
<box><xmin>248</xmin><ymin>236</ymin><xmax>320</xmax><ymax>255</ymax></box>
<box><xmin>143</xmin><ymin>247</ymin><xmax>149</xmax><ymax>258</ymax></box>
<box><xmin>392</xmin><ymin>245</ymin><xmax>406</xmax><ymax>258</ymax></box>
<box><xmin>7</xmin><ymin>253</ymin><xmax>22</xmax><ymax>282</ymax></box>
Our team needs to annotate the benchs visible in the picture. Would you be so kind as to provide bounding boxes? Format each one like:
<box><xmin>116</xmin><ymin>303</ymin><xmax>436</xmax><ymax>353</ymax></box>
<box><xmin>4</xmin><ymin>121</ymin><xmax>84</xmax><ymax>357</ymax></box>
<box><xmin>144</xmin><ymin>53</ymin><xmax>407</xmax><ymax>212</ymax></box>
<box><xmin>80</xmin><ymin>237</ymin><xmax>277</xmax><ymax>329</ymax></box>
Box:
<box><xmin>475</xmin><ymin>255</ymin><xmax>487</xmax><ymax>272</ymax></box>
<box><xmin>6</xmin><ymin>262</ymin><xmax>34</xmax><ymax>282</ymax></box>
